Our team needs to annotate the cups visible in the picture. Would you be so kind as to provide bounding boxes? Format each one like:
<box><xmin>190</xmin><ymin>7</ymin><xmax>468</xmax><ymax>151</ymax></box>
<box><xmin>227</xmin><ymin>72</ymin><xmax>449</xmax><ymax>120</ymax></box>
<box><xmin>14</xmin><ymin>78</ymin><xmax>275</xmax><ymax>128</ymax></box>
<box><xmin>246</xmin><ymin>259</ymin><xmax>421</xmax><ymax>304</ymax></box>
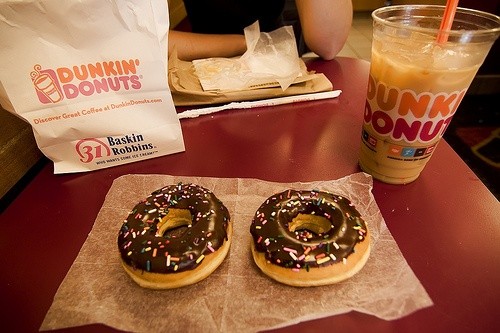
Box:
<box><xmin>358</xmin><ymin>4</ymin><xmax>500</xmax><ymax>184</ymax></box>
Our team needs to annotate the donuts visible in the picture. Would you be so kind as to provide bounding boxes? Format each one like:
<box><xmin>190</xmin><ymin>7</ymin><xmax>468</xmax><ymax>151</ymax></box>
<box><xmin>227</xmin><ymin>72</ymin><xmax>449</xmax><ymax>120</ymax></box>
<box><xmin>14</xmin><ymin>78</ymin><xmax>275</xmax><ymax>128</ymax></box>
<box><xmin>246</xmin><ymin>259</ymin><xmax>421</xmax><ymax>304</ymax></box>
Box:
<box><xmin>117</xmin><ymin>182</ymin><xmax>232</xmax><ymax>289</ymax></box>
<box><xmin>250</xmin><ymin>189</ymin><xmax>371</xmax><ymax>288</ymax></box>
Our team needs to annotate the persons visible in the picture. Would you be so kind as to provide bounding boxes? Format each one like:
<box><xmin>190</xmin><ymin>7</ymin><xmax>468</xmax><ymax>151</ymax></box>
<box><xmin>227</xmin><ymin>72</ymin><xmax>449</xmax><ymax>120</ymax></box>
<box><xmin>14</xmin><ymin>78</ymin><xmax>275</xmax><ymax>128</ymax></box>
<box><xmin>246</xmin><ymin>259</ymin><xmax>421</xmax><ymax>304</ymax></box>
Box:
<box><xmin>168</xmin><ymin>0</ymin><xmax>354</xmax><ymax>61</ymax></box>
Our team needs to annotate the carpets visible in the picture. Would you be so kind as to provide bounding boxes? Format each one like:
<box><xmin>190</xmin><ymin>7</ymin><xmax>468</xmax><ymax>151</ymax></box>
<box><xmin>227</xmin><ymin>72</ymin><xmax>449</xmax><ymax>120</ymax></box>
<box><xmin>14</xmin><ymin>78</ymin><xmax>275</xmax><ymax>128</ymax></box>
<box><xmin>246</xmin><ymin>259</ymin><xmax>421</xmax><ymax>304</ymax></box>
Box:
<box><xmin>440</xmin><ymin>89</ymin><xmax>500</xmax><ymax>203</ymax></box>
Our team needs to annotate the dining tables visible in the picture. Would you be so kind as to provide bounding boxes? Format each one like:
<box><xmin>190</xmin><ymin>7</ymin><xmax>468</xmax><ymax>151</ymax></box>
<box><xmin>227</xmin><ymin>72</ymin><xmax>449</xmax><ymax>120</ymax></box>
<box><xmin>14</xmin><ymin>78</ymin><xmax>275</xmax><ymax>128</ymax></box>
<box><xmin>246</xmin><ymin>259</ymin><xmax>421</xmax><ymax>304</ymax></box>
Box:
<box><xmin>1</xmin><ymin>56</ymin><xmax>500</xmax><ymax>333</ymax></box>
